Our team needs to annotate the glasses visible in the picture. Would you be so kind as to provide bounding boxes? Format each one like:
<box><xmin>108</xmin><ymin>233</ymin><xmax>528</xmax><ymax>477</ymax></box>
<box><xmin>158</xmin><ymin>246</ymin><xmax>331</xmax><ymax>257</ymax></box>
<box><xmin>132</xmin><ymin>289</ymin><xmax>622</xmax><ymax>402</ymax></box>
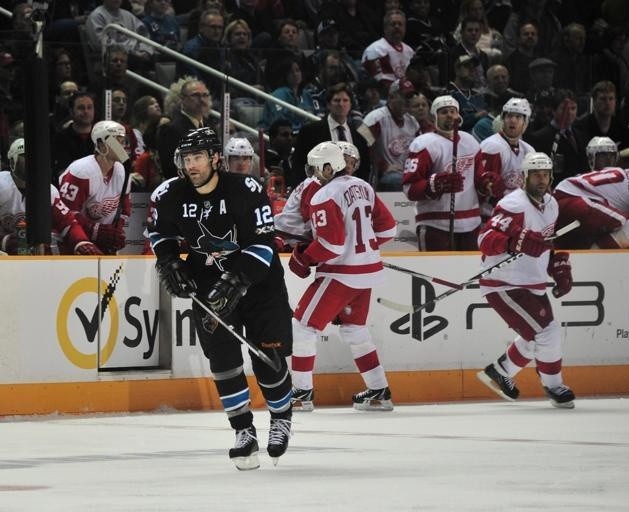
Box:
<box><xmin>184</xmin><ymin>93</ymin><xmax>210</xmax><ymax>98</ymax></box>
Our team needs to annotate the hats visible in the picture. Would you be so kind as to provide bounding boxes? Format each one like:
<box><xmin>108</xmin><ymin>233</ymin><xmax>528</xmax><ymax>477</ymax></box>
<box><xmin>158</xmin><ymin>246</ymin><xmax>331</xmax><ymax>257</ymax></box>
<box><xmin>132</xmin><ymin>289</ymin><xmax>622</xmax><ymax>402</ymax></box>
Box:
<box><xmin>388</xmin><ymin>78</ymin><xmax>419</xmax><ymax>97</ymax></box>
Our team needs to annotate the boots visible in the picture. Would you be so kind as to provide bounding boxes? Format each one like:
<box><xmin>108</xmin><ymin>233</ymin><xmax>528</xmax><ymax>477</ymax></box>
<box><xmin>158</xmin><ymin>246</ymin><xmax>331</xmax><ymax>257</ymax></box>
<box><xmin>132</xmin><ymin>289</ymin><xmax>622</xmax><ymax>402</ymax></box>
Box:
<box><xmin>289</xmin><ymin>385</ymin><xmax>314</xmax><ymax>401</ymax></box>
<box><xmin>232</xmin><ymin>423</ymin><xmax>259</xmax><ymax>458</ymax></box>
<box><xmin>266</xmin><ymin>403</ymin><xmax>293</xmax><ymax>457</ymax></box>
<box><xmin>353</xmin><ymin>386</ymin><xmax>391</xmax><ymax>403</ymax></box>
<box><xmin>484</xmin><ymin>352</ymin><xmax>521</xmax><ymax>399</ymax></box>
<box><xmin>543</xmin><ymin>385</ymin><xmax>576</xmax><ymax>403</ymax></box>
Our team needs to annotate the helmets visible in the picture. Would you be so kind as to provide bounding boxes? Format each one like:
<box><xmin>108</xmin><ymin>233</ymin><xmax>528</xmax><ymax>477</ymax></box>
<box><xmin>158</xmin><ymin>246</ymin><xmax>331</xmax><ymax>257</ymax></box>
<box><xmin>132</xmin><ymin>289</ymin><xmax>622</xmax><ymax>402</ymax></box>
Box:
<box><xmin>519</xmin><ymin>152</ymin><xmax>555</xmax><ymax>191</ymax></box>
<box><xmin>304</xmin><ymin>140</ymin><xmax>347</xmax><ymax>181</ymax></box>
<box><xmin>334</xmin><ymin>140</ymin><xmax>361</xmax><ymax>171</ymax></box>
<box><xmin>586</xmin><ymin>135</ymin><xmax>619</xmax><ymax>171</ymax></box>
<box><xmin>7</xmin><ymin>136</ymin><xmax>25</xmax><ymax>173</ymax></box>
<box><xmin>90</xmin><ymin>120</ymin><xmax>126</xmax><ymax>150</ymax></box>
<box><xmin>501</xmin><ymin>97</ymin><xmax>532</xmax><ymax>118</ymax></box>
<box><xmin>429</xmin><ymin>93</ymin><xmax>460</xmax><ymax>117</ymax></box>
<box><xmin>178</xmin><ymin>126</ymin><xmax>222</xmax><ymax>160</ymax></box>
<box><xmin>224</xmin><ymin>137</ymin><xmax>254</xmax><ymax>159</ymax></box>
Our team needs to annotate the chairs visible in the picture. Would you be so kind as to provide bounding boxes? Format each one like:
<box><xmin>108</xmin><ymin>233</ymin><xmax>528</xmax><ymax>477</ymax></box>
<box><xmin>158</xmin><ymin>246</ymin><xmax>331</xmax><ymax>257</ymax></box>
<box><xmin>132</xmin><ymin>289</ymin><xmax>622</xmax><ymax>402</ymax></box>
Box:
<box><xmin>0</xmin><ymin>1</ymin><xmax>629</xmax><ymax>190</ymax></box>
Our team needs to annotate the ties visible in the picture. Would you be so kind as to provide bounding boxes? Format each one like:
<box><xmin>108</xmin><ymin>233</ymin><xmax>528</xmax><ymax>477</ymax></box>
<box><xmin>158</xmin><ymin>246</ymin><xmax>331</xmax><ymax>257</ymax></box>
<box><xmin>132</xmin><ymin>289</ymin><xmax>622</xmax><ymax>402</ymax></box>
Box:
<box><xmin>335</xmin><ymin>123</ymin><xmax>347</xmax><ymax>140</ymax></box>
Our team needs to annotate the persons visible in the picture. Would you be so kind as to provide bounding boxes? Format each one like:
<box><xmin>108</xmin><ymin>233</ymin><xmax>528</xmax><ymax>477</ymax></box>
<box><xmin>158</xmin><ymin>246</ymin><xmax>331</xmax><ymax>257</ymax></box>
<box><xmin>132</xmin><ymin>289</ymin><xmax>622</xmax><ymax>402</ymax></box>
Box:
<box><xmin>145</xmin><ymin>125</ymin><xmax>294</xmax><ymax>458</ymax></box>
<box><xmin>288</xmin><ymin>141</ymin><xmax>397</xmax><ymax>403</ymax></box>
<box><xmin>476</xmin><ymin>152</ymin><xmax>576</xmax><ymax>402</ymax></box>
<box><xmin>0</xmin><ymin>0</ymin><xmax>629</xmax><ymax>256</ymax></box>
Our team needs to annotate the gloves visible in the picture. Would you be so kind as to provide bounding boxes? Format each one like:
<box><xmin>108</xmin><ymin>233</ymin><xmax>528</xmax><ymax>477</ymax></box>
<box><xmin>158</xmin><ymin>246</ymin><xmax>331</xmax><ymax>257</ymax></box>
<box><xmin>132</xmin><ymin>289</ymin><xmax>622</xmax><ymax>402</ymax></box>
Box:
<box><xmin>288</xmin><ymin>242</ymin><xmax>311</xmax><ymax>279</ymax></box>
<box><xmin>153</xmin><ymin>254</ymin><xmax>198</xmax><ymax>298</ymax></box>
<box><xmin>96</xmin><ymin>223</ymin><xmax>128</xmax><ymax>253</ymax></box>
<box><xmin>507</xmin><ymin>226</ymin><xmax>553</xmax><ymax>257</ymax></box>
<box><xmin>548</xmin><ymin>250</ymin><xmax>573</xmax><ymax>298</ymax></box>
<box><xmin>205</xmin><ymin>269</ymin><xmax>248</xmax><ymax>317</ymax></box>
<box><xmin>477</xmin><ymin>170</ymin><xmax>506</xmax><ymax>199</ymax></box>
<box><xmin>424</xmin><ymin>172</ymin><xmax>465</xmax><ymax>198</ymax></box>
<box><xmin>73</xmin><ymin>239</ymin><xmax>105</xmax><ymax>255</ymax></box>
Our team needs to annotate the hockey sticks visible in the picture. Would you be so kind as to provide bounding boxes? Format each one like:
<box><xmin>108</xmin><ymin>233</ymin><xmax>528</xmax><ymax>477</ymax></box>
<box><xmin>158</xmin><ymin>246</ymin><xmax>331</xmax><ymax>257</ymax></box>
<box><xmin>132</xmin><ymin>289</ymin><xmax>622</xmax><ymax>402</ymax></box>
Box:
<box><xmin>222</xmin><ymin>48</ymin><xmax>231</xmax><ymax>168</ymax></box>
<box><xmin>106</xmin><ymin>135</ymin><xmax>131</xmax><ymax>226</ymax></box>
<box><xmin>184</xmin><ymin>286</ymin><xmax>282</xmax><ymax>373</ymax></box>
<box><xmin>377</xmin><ymin>220</ymin><xmax>582</xmax><ymax>313</ymax></box>
<box><xmin>273</xmin><ymin>229</ymin><xmax>467</xmax><ymax>290</ymax></box>
